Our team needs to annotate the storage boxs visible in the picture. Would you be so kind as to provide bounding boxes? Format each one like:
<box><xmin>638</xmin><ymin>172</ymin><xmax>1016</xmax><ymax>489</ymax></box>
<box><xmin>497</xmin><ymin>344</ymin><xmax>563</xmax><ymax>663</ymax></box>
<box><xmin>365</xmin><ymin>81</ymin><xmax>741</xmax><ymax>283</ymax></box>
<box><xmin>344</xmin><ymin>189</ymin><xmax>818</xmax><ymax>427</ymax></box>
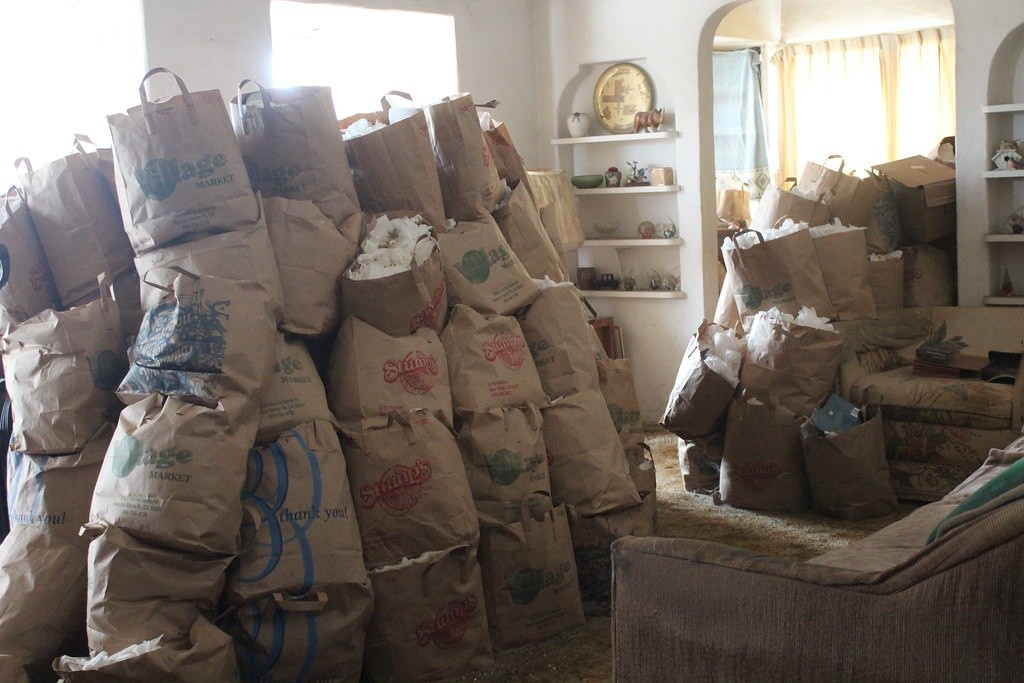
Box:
<box><xmin>872</xmin><ymin>154</ymin><xmax>956</xmax><ymax>249</ymax></box>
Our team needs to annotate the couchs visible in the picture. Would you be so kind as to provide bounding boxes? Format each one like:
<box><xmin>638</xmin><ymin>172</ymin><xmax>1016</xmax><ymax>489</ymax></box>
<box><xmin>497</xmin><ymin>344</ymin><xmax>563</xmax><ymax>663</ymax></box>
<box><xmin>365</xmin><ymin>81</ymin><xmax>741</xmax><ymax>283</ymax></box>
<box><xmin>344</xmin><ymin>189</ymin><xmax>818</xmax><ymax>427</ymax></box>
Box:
<box><xmin>834</xmin><ymin>305</ymin><xmax>1024</xmax><ymax>503</ymax></box>
<box><xmin>611</xmin><ymin>435</ymin><xmax>1024</xmax><ymax>682</ymax></box>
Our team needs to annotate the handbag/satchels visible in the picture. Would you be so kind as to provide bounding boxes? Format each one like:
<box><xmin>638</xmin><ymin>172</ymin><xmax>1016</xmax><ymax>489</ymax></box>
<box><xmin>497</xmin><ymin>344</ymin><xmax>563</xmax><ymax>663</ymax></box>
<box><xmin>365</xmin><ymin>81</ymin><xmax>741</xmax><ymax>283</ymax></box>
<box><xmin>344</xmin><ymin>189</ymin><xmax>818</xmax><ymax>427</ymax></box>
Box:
<box><xmin>661</xmin><ymin>155</ymin><xmax>909</xmax><ymax>521</ymax></box>
<box><xmin>0</xmin><ymin>68</ymin><xmax>661</xmax><ymax>683</ymax></box>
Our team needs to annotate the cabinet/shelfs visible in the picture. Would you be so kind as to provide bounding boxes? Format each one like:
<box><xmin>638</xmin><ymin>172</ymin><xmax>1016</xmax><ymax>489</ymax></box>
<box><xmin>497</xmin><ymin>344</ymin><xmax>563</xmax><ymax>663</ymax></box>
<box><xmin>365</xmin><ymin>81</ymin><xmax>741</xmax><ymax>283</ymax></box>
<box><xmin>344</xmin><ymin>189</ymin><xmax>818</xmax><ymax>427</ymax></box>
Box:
<box><xmin>550</xmin><ymin>131</ymin><xmax>685</xmax><ymax>300</ymax></box>
<box><xmin>979</xmin><ymin>102</ymin><xmax>1024</xmax><ymax>306</ymax></box>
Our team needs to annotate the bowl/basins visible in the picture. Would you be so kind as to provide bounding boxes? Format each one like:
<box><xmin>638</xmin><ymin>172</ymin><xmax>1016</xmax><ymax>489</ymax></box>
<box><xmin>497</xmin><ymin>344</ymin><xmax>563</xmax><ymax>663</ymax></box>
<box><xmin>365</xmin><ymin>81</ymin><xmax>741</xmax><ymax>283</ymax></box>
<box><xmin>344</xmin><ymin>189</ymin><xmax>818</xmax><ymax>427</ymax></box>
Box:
<box><xmin>571</xmin><ymin>175</ymin><xmax>603</xmax><ymax>188</ymax></box>
